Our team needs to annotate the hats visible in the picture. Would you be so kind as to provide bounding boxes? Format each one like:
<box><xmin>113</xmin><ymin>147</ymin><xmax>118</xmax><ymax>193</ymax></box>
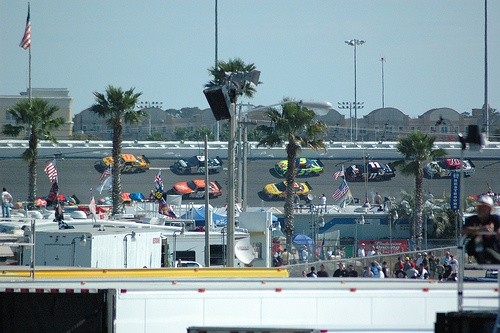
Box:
<box><xmin>475</xmin><ymin>195</ymin><xmax>496</xmax><ymax>209</ymax></box>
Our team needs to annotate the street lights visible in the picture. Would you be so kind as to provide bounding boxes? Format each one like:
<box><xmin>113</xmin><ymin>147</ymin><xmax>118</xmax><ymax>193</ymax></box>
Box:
<box><xmin>355</xmin><ymin>215</ymin><xmax>366</xmax><ymax>258</ymax></box>
<box><xmin>136</xmin><ymin>102</ymin><xmax>162</xmax><ymax>136</ymax></box>
<box><xmin>71</xmin><ymin>234</ymin><xmax>87</xmax><ymax>267</ymax></box>
<box><xmin>269</xmin><ymin>221</ymin><xmax>282</xmax><ymax>268</ymax></box>
<box><xmin>344</xmin><ymin>38</ymin><xmax>366</xmax><ymax>141</ymax></box>
<box><xmin>337</xmin><ymin>101</ymin><xmax>364</xmax><ymax>142</ymax></box>
<box><xmin>423</xmin><ymin>210</ymin><xmax>435</xmax><ymax>250</ymax></box>
<box><xmin>388</xmin><ymin>210</ymin><xmax>399</xmax><ymax>255</ymax></box>
<box><xmin>172</xmin><ymin>228</ymin><xmax>185</xmax><ymax>267</ymax></box>
<box><xmin>124</xmin><ymin>231</ymin><xmax>137</xmax><ymax>269</ymax></box>
<box><xmin>316</xmin><ymin>217</ymin><xmax>325</xmax><ymax>243</ymax></box>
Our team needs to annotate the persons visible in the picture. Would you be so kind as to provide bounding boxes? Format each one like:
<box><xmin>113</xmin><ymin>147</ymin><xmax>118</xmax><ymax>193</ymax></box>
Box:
<box><xmin>428</xmin><ymin>192</ymin><xmax>433</xmax><ymax>199</ymax></box>
<box><xmin>320</xmin><ymin>194</ymin><xmax>326</xmax><ymax>206</ymax></box>
<box><xmin>1</xmin><ymin>187</ymin><xmax>13</xmax><ymax>218</ymax></box>
<box><xmin>463</xmin><ymin>194</ymin><xmax>500</xmax><ymax>264</ymax></box>
<box><xmin>374</xmin><ymin>192</ymin><xmax>382</xmax><ymax>204</ymax></box>
<box><xmin>393</xmin><ymin>251</ymin><xmax>459</xmax><ymax>280</ymax></box>
<box><xmin>294</xmin><ymin>192</ymin><xmax>300</xmax><ymax>203</ymax></box>
<box><xmin>306</xmin><ymin>260</ymin><xmax>389</xmax><ymax>277</ymax></box>
<box><xmin>273</xmin><ymin>243</ymin><xmax>378</xmax><ymax>267</ymax></box>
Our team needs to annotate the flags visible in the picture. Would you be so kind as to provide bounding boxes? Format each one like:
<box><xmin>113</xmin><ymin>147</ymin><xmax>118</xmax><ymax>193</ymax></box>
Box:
<box><xmin>153</xmin><ymin>169</ymin><xmax>163</xmax><ymax>187</ymax></box>
<box><xmin>44</xmin><ymin>158</ymin><xmax>57</xmax><ymax>183</ymax></box>
<box><xmin>96</xmin><ymin>164</ymin><xmax>111</xmax><ymax>194</ymax></box>
<box><xmin>156</xmin><ymin>197</ymin><xmax>177</xmax><ymax>217</ymax></box>
<box><xmin>17</xmin><ymin>4</ymin><xmax>31</xmax><ymax>50</ymax></box>
<box><xmin>338</xmin><ymin>191</ymin><xmax>353</xmax><ymax>209</ymax></box>
<box><xmin>331</xmin><ymin>180</ymin><xmax>349</xmax><ymax>201</ymax></box>
<box><xmin>332</xmin><ymin>170</ymin><xmax>345</xmax><ymax>180</ymax></box>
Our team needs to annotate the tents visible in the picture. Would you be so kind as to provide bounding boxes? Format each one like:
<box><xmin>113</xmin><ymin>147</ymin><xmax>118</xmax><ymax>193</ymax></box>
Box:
<box><xmin>180</xmin><ymin>206</ymin><xmax>241</xmax><ymax>227</ymax></box>
<box><xmin>290</xmin><ymin>233</ymin><xmax>314</xmax><ymax>244</ymax></box>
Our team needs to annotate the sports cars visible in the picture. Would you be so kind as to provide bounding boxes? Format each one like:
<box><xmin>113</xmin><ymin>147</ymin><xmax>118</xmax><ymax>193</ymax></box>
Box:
<box><xmin>274</xmin><ymin>157</ymin><xmax>324</xmax><ymax>178</ymax></box>
<box><xmin>263</xmin><ymin>180</ymin><xmax>313</xmax><ymax>201</ymax></box>
<box><xmin>424</xmin><ymin>158</ymin><xmax>475</xmax><ymax>179</ymax></box>
<box><xmin>171</xmin><ymin>179</ymin><xmax>223</xmax><ymax>200</ymax></box>
<box><xmin>344</xmin><ymin>161</ymin><xmax>397</xmax><ymax>182</ymax></box>
<box><xmin>100</xmin><ymin>153</ymin><xmax>150</xmax><ymax>174</ymax></box>
<box><xmin>172</xmin><ymin>155</ymin><xmax>224</xmax><ymax>175</ymax></box>
